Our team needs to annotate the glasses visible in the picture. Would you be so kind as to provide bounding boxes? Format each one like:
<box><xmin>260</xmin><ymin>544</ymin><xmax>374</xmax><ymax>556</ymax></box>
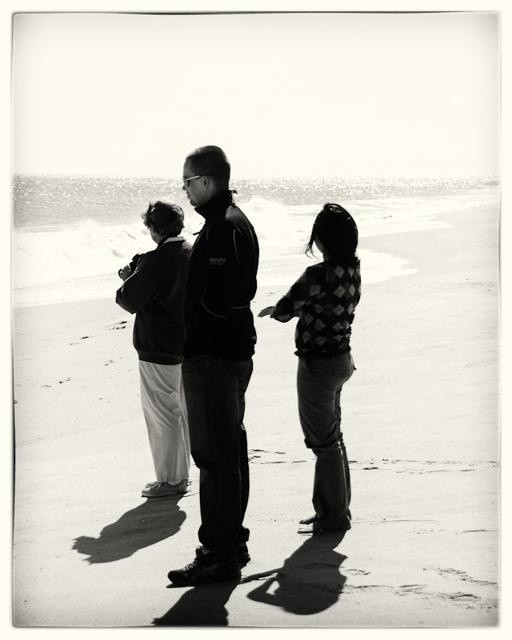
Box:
<box><xmin>184</xmin><ymin>175</ymin><xmax>202</xmax><ymax>187</ymax></box>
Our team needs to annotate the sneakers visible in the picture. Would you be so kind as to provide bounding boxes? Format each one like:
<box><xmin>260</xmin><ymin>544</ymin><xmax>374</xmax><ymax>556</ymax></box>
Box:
<box><xmin>142</xmin><ymin>479</ymin><xmax>188</xmax><ymax>496</ymax></box>
<box><xmin>299</xmin><ymin>509</ymin><xmax>351</xmax><ymax>534</ymax></box>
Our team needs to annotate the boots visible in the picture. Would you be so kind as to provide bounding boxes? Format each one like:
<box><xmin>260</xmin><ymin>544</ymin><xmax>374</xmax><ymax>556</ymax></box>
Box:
<box><xmin>169</xmin><ymin>542</ymin><xmax>250</xmax><ymax>585</ymax></box>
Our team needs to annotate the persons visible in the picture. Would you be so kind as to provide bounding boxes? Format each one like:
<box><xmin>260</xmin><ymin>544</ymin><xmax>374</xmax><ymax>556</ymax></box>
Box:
<box><xmin>257</xmin><ymin>203</ymin><xmax>361</xmax><ymax>534</ymax></box>
<box><xmin>167</xmin><ymin>146</ymin><xmax>259</xmax><ymax>586</ymax></box>
<box><xmin>115</xmin><ymin>202</ymin><xmax>193</xmax><ymax>497</ymax></box>
